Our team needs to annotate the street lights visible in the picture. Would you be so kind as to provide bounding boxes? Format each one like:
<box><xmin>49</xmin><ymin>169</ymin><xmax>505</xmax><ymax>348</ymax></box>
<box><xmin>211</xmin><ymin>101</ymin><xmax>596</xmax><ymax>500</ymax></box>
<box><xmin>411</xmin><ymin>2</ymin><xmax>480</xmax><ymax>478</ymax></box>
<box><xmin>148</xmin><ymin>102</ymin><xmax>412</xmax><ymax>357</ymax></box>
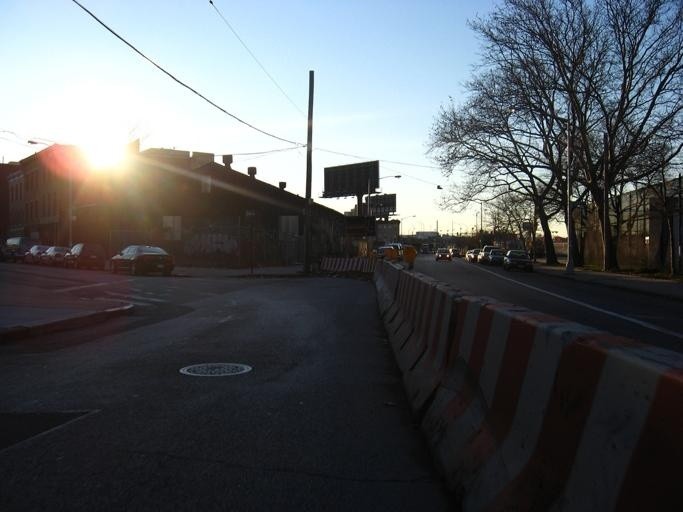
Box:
<box><xmin>365</xmin><ymin>173</ymin><xmax>402</xmax><ymax>257</ymax></box>
<box><xmin>25</xmin><ymin>139</ymin><xmax>73</xmax><ymax>252</ymax></box>
<box><xmin>398</xmin><ymin>213</ymin><xmax>415</xmax><ymax>239</ymax></box>
<box><xmin>459</xmin><ymin>196</ymin><xmax>485</xmax><ymax>252</ymax></box>
<box><xmin>506</xmin><ymin>99</ymin><xmax>578</xmax><ymax>275</ymax></box>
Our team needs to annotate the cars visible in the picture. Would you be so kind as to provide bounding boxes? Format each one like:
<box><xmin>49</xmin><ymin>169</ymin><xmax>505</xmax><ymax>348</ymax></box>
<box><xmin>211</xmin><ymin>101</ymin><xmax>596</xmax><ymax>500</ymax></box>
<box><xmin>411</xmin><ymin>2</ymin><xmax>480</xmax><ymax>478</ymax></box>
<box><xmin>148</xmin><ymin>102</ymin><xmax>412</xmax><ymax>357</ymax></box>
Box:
<box><xmin>372</xmin><ymin>237</ymin><xmax>534</xmax><ymax>277</ymax></box>
<box><xmin>0</xmin><ymin>233</ymin><xmax>176</xmax><ymax>279</ymax></box>
